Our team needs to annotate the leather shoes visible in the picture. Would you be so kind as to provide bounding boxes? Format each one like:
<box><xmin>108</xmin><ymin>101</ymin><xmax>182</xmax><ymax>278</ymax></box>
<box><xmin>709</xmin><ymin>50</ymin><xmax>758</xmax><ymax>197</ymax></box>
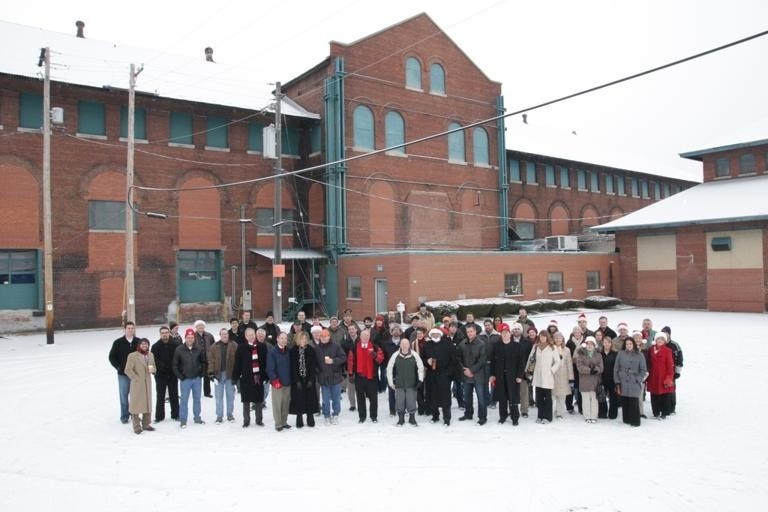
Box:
<box><xmin>231</xmin><ymin>380</ymin><xmax>237</xmax><ymax>385</ymax></box>
<box><xmin>307</xmin><ymin>381</ymin><xmax>312</xmax><ymax>389</ymax></box>
<box><xmin>271</xmin><ymin>378</ymin><xmax>282</xmax><ymax>389</ymax></box>
<box><xmin>180</xmin><ymin>374</ymin><xmax>185</xmax><ymax>380</ymax></box>
<box><xmin>296</xmin><ymin>381</ymin><xmax>303</xmax><ymax>393</ymax></box>
<box><xmin>414</xmin><ymin>381</ymin><xmax>422</xmax><ymax>392</ymax></box>
<box><xmin>211</xmin><ymin>376</ymin><xmax>219</xmax><ymax>386</ymax></box>
<box><xmin>590</xmin><ymin>367</ymin><xmax>598</xmax><ymax>375</ymax></box>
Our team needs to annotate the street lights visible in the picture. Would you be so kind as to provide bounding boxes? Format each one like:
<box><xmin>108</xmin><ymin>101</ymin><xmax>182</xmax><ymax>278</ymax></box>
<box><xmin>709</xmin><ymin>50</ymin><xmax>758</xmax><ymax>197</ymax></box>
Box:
<box><xmin>527</xmin><ymin>344</ymin><xmax>538</xmax><ymax>373</ymax></box>
<box><xmin>596</xmin><ymin>383</ymin><xmax>607</xmax><ymax>403</ymax></box>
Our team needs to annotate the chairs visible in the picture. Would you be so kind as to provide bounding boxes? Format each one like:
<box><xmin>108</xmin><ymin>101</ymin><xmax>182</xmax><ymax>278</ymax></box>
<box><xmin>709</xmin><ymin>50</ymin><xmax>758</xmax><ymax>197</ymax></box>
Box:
<box><xmin>120</xmin><ymin>393</ymin><xmax>339</xmax><ymax>435</ymax></box>
<box><xmin>388</xmin><ymin>399</ymin><xmax>677</xmax><ymax>428</ymax></box>
<box><xmin>348</xmin><ymin>404</ymin><xmax>378</xmax><ymax>425</ymax></box>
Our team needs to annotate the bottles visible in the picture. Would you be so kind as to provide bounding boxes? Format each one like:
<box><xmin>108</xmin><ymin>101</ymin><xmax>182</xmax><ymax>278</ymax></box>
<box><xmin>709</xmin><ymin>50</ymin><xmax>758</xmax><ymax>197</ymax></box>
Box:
<box><xmin>442</xmin><ymin>316</ymin><xmax>451</xmax><ymax>322</ymax></box>
<box><xmin>310</xmin><ymin>325</ymin><xmax>322</xmax><ymax>333</ymax></box>
<box><xmin>549</xmin><ymin>320</ymin><xmax>563</xmax><ymax>338</ymax></box>
<box><xmin>419</xmin><ymin>303</ymin><xmax>427</xmax><ymax>307</ymax></box>
<box><xmin>184</xmin><ymin>329</ymin><xmax>195</xmax><ymax>337</ymax></box>
<box><xmin>329</xmin><ymin>316</ymin><xmax>338</xmax><ymax>323</ymax></box>
<box><xmin>632</xmin><ymin>329</ymin><xmax>642</xmax><ymax>337</ymax></box>
<box><xmin>264</xmin><ymin>311</ymin><xmax>273</xmax><ymax>318</ymax></box>
<box><xmin>343</xmin><ymin>308</ymin><xmax>353</xmax><ymax>318</ymax></box>
<box><xmin>577</xmin><ymin>313</ymin><xmax>587</xmax><ymax>322</ymax></box>
<box><xmin>661</xmin><ymin>326</ymin><xmax>672</xmax><ymax>334</ymax></box>
<box><xmin>294</xmin><ymin>320</ymin><xmax>301</xmax><ymax>326</ymax></box>
<box><xmin>573</xmin><ymin>325</ymin><xmax>581</xmax><ymax>333</ymax></box>
<box><xmin>654</xmin><ymin>332</ymin><xmax>668</xmax><ymax>342</ymax></box>
<box><xmin>389</xmin><ymin>323</ymin><xmax>403</xmax><ymax>336</ymax></box>
<box><xmin>193</xmin><ymin>320</ymin><xmax>207</xmax><ymax>329</ymax></box>
<box><xmin>497</xmin><ymin>323</ymin><xmax>510</xmax><ymax>332</ymax></box>
<box><xmin>584</xmin><ymin>335</ymin><xmax>597</xmax><ymax>345</ymax></box>
<box><xmin>376</xmin><ymin>314</ymin><xmax>385</xmax><ymax>321</ymax></box>
<box><xmin>169</xmin><ymin>320</ymin><xmax>178</xmax><ymax>330</ymax></box>
<box><xmin>428</xmin><ymin>327</ymin><xmax>448</xmax><ymax>338</ymax></box>
<box><xmin>512</xmin><ymin>306</ymin><xmax>538</xmax><ymax>333</ymax></box>
<box><xmin>617</xmin><ymin>322</ymin><xmax>628</xmax><ymax>331</ymax></box>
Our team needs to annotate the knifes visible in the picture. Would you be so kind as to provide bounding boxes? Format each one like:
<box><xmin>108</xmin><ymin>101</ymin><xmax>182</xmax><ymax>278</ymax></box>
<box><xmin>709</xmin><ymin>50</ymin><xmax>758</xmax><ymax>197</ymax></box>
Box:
<box><xmin>544</xmin><ymin>232</ymin><xmax>579</xmax><ymax>252</ymax></box>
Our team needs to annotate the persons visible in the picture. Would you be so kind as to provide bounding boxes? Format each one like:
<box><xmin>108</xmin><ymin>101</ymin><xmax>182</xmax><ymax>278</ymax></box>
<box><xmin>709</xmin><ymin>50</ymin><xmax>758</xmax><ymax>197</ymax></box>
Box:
<box><xmin>238</xmin><ymin>310</ymin><xmax>257</xmax><ymax>333</ymax></box>
<box><xmin>384</xmin><ymin>304</ymin><xmax>535</xmax><ymax>427</ymax></box>
<box><xmin>266</xmin><ymin>308</ymin><xmax>391</xmax><ymax>432</ymax></box>
<box><xmin>259</xmin><ymin>311</ymin><xmax>280</xmax><ymax>344</ymax></box>
<box><xmin>525</xmin><ymin>313</ymin><xmax>682</xmax><ymax>427</ymax></box>
<box><xmin>109</xmin><ymin>317</ymin><xmax>275</xmax><ymax>433</ymax></box>
